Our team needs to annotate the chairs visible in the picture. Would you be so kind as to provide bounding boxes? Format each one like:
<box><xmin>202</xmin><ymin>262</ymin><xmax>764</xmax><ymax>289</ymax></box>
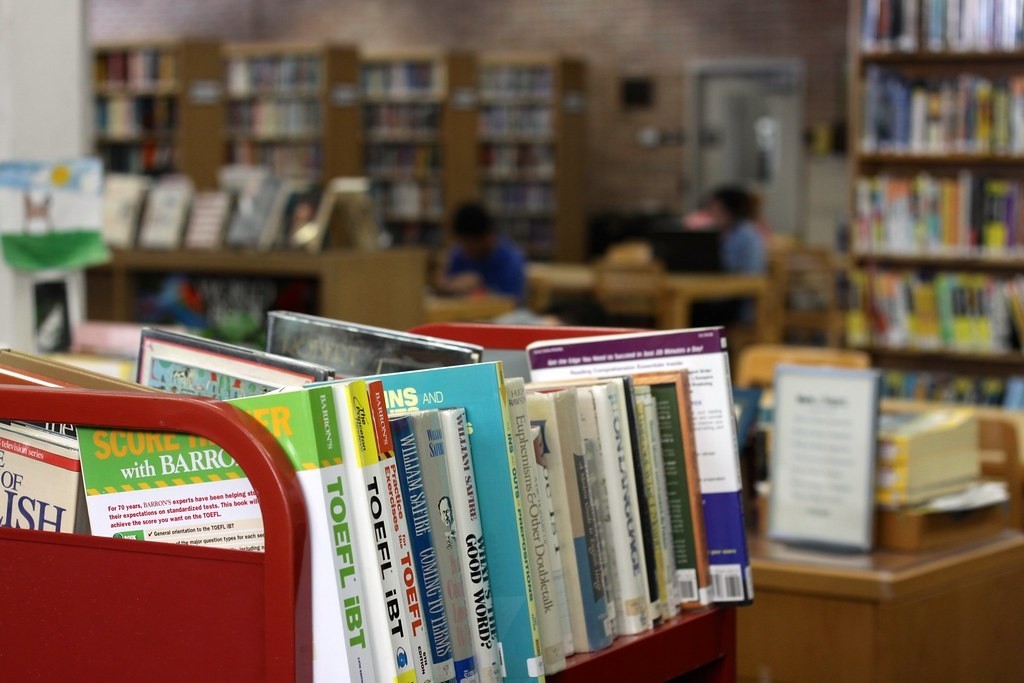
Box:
<box><xmin>769</xmin><ymin>247</ymin><xmax>849</xmax><ymax>351</ymax></box>
<box><xmin>733</xmin><ymin>346</ymin><xmax>867</xmax><ymax>390</ymax></box>
<box><xmin>976</xmin><ymin>410</ymin><xmax>1024</xmax><ymax>528</ymax></box>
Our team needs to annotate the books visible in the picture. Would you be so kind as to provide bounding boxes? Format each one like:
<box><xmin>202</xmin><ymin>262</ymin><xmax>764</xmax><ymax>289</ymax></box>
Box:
<box><xmin>363</xmin><ymin>59</ymin><xmax>442</xmax><ymax>245</ymax></box>
<box><xmin>101</xmin><ymin>161</ymin><xmax>268</xmax><ymax>250</ymax></box>
<box><xmin>222</xmin><ymin>53</ymin><xmax>320</xmax><ymax>172</ymax></box>
<box><xmin>476</xmin><ymin>62</ymin><xmax>550</xmax><ymax>255</ymax></box>
<box><xmin>0</xmin><ymin>308</ymin><xmax>757</xmax><ymax>683</ymax></box>
<box><xmin>845</xmin><ymin>1</ymin><xmax>1023</xmax><ymax>409</ymax></box>
<box><xmin>95</xmin><ymin>50</ymin><xmax>172</xmax><ymax>177</ymax></box>
<box><xmin>767</xmin><ymin>406</ymin><xmax>983</xmax><ymax>510</ymax></box>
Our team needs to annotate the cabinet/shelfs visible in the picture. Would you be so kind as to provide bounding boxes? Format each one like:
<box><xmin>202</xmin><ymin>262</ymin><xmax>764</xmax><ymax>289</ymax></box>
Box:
<box><xmin>360</xmin><ymin>49</ymin><xmax>480</xmax><ymax>252</ymax></box>
<box><xmin>107</xmin><ymin>243</ymin><xmax>427</xmax><ymax>332</ymax></box>
<box><xmin>222</xmin><ymin>39</ymin><xmax>361</xmax><ymax>179</ymax></box>
<box><xmin>89</xmin><ymin>37</ymin><xmax>226</xmax><ymax>192</ymax></box>
<box><xmin>481</xmin><ymin>54</ymin><xmax>587</xmax><ymax>267</ymax></box>
<box><xmin>848</xmin><ymin>0</ymin><xmax>1024</xmax><ymax>408</ymax></box>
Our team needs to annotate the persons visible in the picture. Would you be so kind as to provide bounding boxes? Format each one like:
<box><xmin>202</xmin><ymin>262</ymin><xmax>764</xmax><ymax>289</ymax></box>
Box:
<box><xmin>436</xmin><ymin>203</ymin><xmax>526</xmax><ymax>305</ymax></box>
<box><xmin>677</xmin><ymin>189</ymin><xmax>767</xmax><ymax>331</ymax></box>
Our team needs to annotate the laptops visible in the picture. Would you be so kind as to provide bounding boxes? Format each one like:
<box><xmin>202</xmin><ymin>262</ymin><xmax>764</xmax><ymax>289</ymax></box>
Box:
<box><xmin>649</xmin><ymin>230</ymin><xmax>724</xmax><ymax>273</ymax></box>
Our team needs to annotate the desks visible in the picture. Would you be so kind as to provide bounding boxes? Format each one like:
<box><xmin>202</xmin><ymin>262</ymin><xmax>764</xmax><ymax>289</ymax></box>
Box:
<box><xmin>527</xmin><ymin>264</ymin><xmax>773</xmax><ymax>345</ymax></box>
<box><xmin>735</xmin><ymin>506</ymin><xmax>1024</xmax><ymax>683</ymax></box>
